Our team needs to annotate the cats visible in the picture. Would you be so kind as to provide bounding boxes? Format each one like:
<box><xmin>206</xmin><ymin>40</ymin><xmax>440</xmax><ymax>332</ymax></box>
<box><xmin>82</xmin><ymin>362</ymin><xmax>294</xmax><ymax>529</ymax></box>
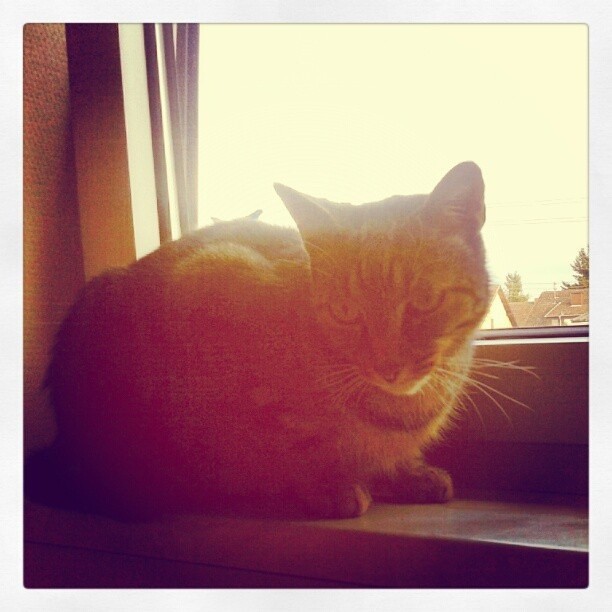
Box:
<box><xmin>23</xmin><ymin>158</ymin><xmax>546</xmax><ymax>526</ymax></box>
